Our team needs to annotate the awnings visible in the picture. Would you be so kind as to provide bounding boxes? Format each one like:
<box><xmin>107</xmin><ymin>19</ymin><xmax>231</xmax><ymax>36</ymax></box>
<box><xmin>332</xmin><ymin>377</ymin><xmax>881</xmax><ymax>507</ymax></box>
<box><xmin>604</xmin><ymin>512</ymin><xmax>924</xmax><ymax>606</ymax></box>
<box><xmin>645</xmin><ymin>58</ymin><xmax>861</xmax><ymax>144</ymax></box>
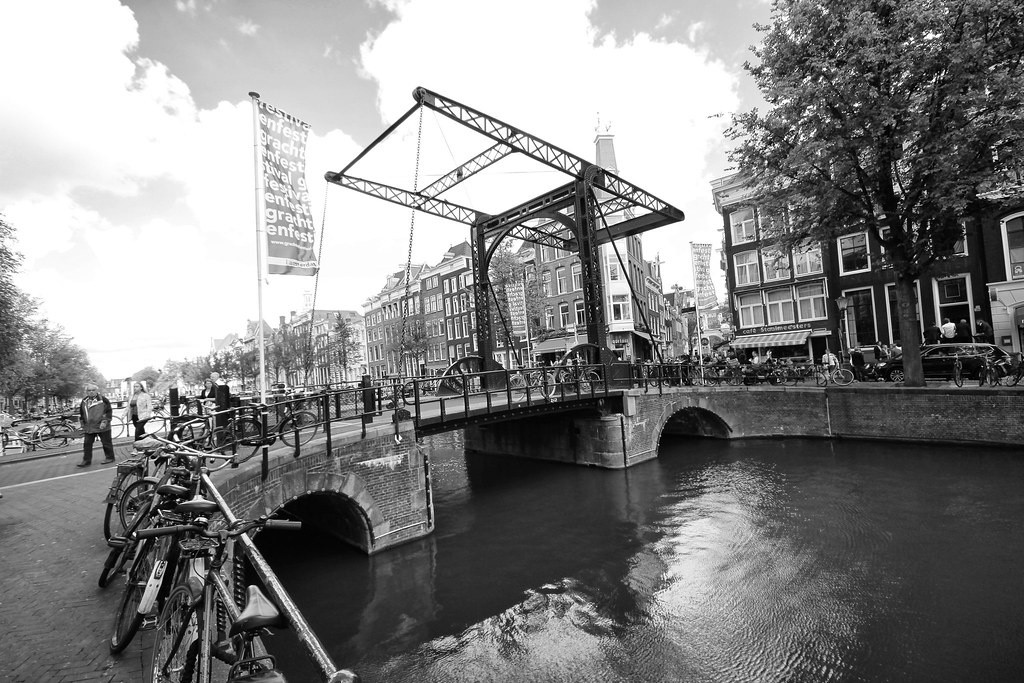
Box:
<box><xmin>1014</xmin><ymin>306</ymin><xmax>1024</xmax><ymax>325</ymax></box>
<box><xmin>712</xmin><ymin>339</ymin><xmax>731</xmax><ymax>350</ymax></box>
<box><xmin>729</xmin><ymin>329</ymin><xmax>812</xmax><ymax>348</ymax></box>
<box><xmin>632</xmin><ymin>331</ymin><xmax>664</xmax><ymax>343</ymax></box>
<box><xmin>530</xmin><ymin>334</ymin><xmax>588</xmax><ymax>355</ymax></box>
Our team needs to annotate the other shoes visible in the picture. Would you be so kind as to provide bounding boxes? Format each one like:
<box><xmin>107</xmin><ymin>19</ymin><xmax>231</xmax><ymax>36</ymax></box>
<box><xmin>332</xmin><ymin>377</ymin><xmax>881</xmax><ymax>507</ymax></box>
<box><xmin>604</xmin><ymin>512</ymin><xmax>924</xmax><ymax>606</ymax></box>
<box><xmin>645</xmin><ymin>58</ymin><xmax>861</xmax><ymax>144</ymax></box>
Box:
<box><xmin>100</xmin><ymin>457</ymin><xmax>115</xmax><ymax>464</ymax></box>
<box><xmin>130</xmin><ymin>447</ymin><xmax>138</xmax><ymax>456</ymax></box>
<box><xmin>137</xmin><ymin>449</ymin><xmax>145</xmax><ymax>454</ymax></box>
<box><xmin>77</xmin><ymin>459</ymin><xmax>91</xmax><ymax>467</ymax></box>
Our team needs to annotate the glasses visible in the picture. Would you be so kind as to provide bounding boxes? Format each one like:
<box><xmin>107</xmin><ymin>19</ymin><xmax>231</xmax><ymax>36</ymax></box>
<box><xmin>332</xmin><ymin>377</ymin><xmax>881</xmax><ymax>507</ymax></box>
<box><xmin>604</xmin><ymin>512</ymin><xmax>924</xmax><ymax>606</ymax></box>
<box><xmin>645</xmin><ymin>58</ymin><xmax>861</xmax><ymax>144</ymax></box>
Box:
<box><xmin>87</xmin><ymin>389</ymin><xmax>96</xmax><ymax>391</ymax></box>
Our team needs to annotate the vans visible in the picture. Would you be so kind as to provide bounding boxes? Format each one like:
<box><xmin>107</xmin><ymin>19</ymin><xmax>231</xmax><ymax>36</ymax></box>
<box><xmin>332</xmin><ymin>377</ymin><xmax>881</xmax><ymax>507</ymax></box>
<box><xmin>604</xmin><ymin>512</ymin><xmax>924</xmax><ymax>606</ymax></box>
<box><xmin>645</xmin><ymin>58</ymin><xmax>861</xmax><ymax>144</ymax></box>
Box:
<box><xmin>875</xmin><ymin>343</ymin><xmax>1011</xmax><ymax>381</ymax></box>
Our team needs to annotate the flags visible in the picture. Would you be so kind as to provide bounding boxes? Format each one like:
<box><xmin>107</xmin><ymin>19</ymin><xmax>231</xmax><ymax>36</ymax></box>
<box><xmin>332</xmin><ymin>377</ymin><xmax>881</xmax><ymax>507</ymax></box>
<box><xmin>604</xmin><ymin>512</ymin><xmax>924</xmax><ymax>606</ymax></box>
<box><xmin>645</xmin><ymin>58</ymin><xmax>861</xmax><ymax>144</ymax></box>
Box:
<box><xmin>504</xmin><ymin>283</ymin><xmax>526</xmax><ymax>337</ymax></box>
<box><xmin>691</xmin><ymin>243</ymin><xmax>719</xmax><ymax>311</ymax></box>
<box><xmin>257</xmin><ymin>99</ymin><xmax>320</xmax><ymax>277</ymax></box>
<box><xmin>700</xmin><ymin>333</ymin><xmax>711</xmax><ymax>354</ymax></box>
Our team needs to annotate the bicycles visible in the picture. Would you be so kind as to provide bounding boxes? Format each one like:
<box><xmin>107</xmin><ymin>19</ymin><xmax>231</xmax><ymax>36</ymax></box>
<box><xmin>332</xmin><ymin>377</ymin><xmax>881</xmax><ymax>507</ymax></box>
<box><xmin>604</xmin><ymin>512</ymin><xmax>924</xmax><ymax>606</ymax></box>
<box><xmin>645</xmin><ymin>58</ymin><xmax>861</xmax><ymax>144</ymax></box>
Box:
<box><xmin>950</xmin><ymin>351</ymin><xmax>1024</xmax><ymax>389</ymax></box>
<box><xmin>503</xmin><ymin>344</ymin><xmax>854</xmax><ymax>399</ymax></box>
<box><xmin>1</xmin><ymin>385</ymin><xmax>318</xmax><ymax>683</ymax></box>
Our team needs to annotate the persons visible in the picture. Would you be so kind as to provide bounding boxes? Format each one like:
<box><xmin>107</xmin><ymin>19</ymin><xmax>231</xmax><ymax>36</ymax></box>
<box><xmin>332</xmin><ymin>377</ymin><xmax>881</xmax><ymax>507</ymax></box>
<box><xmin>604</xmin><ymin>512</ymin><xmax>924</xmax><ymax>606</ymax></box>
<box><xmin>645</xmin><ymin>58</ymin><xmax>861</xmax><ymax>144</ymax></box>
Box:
<box><xmin>1</xmin><ymin>402</ymin><xmax>70</xmax><ymax>425</ymax></box>
<box><xmin>971</xmin><ymin>319</ymin><xmax>993</xmax><ymax>344</ymax></box>
<box><xmin>209</xmin><ymin>372</ymin><xmax>226</xmax><ymax>388</ymax></box>
<box><xmin>940</xmin><ymin>317</ymin><xmax>957</xmax><ymax>344</ymax></box>
<box><xmin>518</xmin><ymin>346</ymin><xmax>806</xmax><ymax>389</ymax></box>
<box><xmin>821</xmin><ymin>348</ymin><xmax>838</xmax><ymax>383</ymax></box>
<box><xmin>233</xmin><ymin>367</ymin><xmax>480</xmax><ymax>405</ymax></box>
<box><xmin>200</xmin><ymin>378</ymin><xmax>216</xmax><ymax>443</ymax></box>
<box><xmin>120</xmin><ymin>382</ymin><xmax>153</xmax><ymax>456</ymax></box>
<box><xmin>272</xmin><ymin>384</ymin><xmax>288</xmax><ymax>420</ymax></box>
<box><xmin>849</xmin><ymin>339</ymin><xmax>903</xmax><ymax>383</ymax></box>
<box><xmin>76</xmin><ymin>384</ymin><xmax>115</xmax><ymax>468</ymax></box>
<box><xmin>922</xmin><ymin>320</ymin><xmax>942</xmax><ymax>345</ymax></box>
<box><xmin>956</xmin><ymin>319</ymin><xmax>972</xmax><ymax>343</ymax></box>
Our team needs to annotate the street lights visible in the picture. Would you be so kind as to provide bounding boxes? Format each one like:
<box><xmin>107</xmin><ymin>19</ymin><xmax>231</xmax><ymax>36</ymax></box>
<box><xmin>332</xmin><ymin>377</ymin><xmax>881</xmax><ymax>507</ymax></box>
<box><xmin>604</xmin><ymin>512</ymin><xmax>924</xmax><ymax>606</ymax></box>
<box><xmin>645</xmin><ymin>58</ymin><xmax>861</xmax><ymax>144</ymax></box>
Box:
<box><xmin>836</xmin><ymin>296</ymin><xmax>853</xmax><ymax>382</ymax></box>
